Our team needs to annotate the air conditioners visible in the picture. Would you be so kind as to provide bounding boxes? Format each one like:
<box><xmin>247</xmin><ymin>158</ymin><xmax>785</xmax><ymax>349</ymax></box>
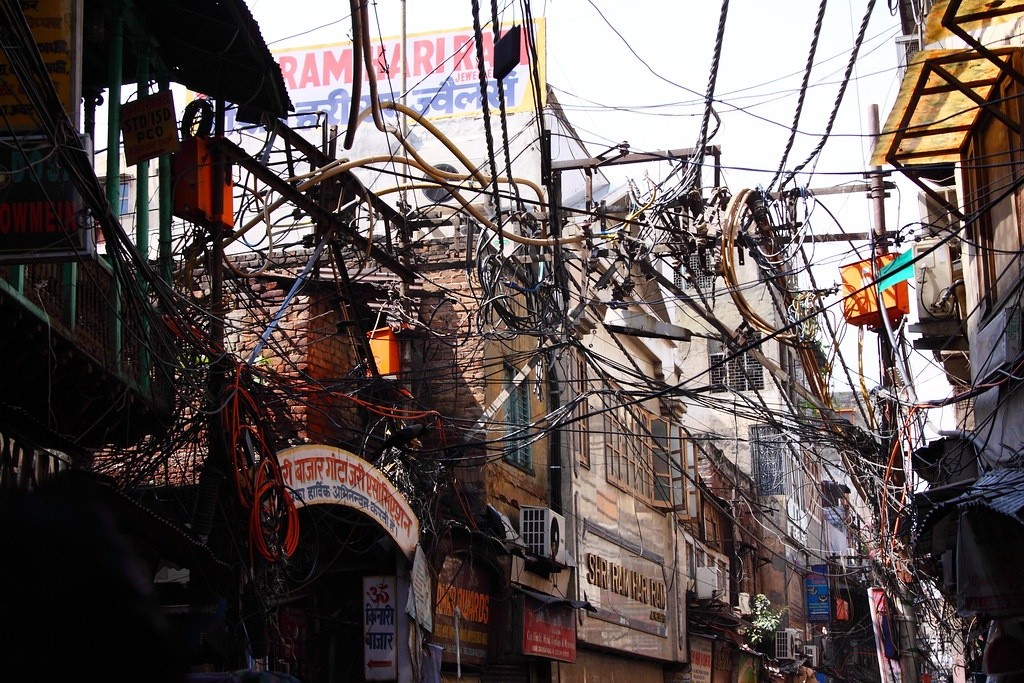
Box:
<box><xmin>912</xmin><ymin>239</ymin><xmax>957</xmax><ymax>338</ymax></box>
<box><xmin>519</xmin><ymin>505</ymin><xmax>566</xmax><ymax>565</ymax></box>
<box><xmin>400</xmin><ymin>155</ymin><xmax>500</xmax><ymax>217</ymax></box>
<box><xmin>775</xmin><ymin>631</ymin><xmax>795</xmax><ymax>661</ymax></box>
<box><xmin>804</xmin><ymin>646</ymin><xmax>820</xmax><ymax>668</ymax></box>
<box><xmin>696</xmin><ymin>567</ymin><xmax>723</xmax><ymax>601</ymax></box>
